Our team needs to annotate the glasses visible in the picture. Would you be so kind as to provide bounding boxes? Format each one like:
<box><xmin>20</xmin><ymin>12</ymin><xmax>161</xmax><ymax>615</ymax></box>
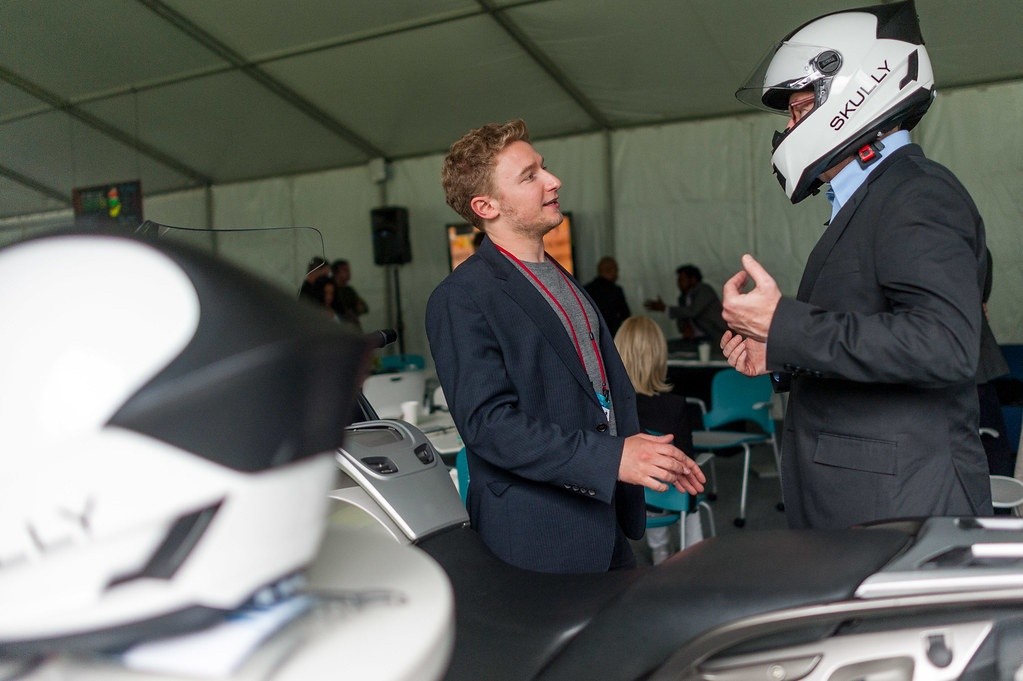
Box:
<box><xmin>789</xmin><ymin>94</ymin><xmax>817</xmax><ymax>122</ymax></box>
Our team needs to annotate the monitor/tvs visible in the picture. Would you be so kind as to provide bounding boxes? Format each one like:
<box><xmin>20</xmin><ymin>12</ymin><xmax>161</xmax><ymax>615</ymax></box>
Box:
<box><xmin>73</xmin><ymin>180</ymin><xmax>143</xmax><ymax>228</ymax></box>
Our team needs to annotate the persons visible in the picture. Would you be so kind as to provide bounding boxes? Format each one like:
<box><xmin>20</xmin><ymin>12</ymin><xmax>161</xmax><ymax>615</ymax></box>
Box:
<box><xmin>582</xmin><ymin>257</ymin><xmax>632</xmax><ymax>338</ymax></box>
<box><xmin>644</xmin><ymin>262</ymin><xmax>726</xmax><ymax>337</ymax></box>
<box><xmin>425</xmin><ymin>121</ymin><xmax>706</xmax><ymax>575</ymax></box>
<box><xmin>300</xmin><ymin>257</ymin><xmax>369</xmax><ymax>334</ymax></box>
<box><xmin>614</xmin><ymin>317</ymin><xmax>696</xmax><ymax>565</ymax></box>
<box><xmin>719</xmin><ymin>1</ymin><xmax>995</xmax><ymax>530</ymax></box>
<box><xmin>976</xmin><ymin>247</ymin><xmax>1013</xmax><ymax>516</ymax></box>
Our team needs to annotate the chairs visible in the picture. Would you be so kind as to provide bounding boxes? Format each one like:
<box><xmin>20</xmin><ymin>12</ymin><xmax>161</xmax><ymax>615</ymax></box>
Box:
<box><xmin>691</xmin><ymin>360</ymin><xmax>789</xmax><ymax>524</ymax></box>
<box><xmin>363</xmin><ymin>371</ymin><xmax>428</xmax><ymax>419</ymax></box>
<box><xmin>641</xmin><ymin>436</ymin><xmax>719</xmax><ymax>551</ymax></box>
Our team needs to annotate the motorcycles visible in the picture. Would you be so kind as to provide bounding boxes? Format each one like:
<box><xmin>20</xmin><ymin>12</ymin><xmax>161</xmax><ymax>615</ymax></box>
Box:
<box><xmin>322</xmin><ymin>323</ymin><xmax>1023</xmax><ymax>681</ymax></box>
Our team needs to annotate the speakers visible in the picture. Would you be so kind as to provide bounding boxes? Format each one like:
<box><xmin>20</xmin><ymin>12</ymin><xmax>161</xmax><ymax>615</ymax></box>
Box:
<box><xmin>371</xmin><ymin>206</ymin><xmax>412</xmax><ymax>267</ymax></box>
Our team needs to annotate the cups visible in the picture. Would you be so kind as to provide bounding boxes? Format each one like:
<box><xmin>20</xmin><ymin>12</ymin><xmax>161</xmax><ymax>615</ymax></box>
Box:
<box><xmin>399</xmin><ymin>401</ymin><xmax>420</xmax><ymax>427</ymax></box>
<box><xmin>698</xmin><ymin>344</ymin><xmax>711</xmax><ymax>362</ymax></box>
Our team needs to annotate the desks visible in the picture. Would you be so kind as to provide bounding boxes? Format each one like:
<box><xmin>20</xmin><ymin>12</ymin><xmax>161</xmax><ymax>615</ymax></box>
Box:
<box><xmin>666</xmin><ymin>357</ymin><xmax>752</xmax><ymax>369</ymax></box>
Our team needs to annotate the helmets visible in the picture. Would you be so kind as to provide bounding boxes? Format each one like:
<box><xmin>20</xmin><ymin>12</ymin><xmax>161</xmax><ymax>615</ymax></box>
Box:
<box><xmin>734</xmin><ymin>1</ymin><xmax>941</xmax><ymax>205</ymax></box>
<box><xmin>0</xmin><ymin>222</ymin><xmax>375</xmax><ymax>664</ymax></box>
